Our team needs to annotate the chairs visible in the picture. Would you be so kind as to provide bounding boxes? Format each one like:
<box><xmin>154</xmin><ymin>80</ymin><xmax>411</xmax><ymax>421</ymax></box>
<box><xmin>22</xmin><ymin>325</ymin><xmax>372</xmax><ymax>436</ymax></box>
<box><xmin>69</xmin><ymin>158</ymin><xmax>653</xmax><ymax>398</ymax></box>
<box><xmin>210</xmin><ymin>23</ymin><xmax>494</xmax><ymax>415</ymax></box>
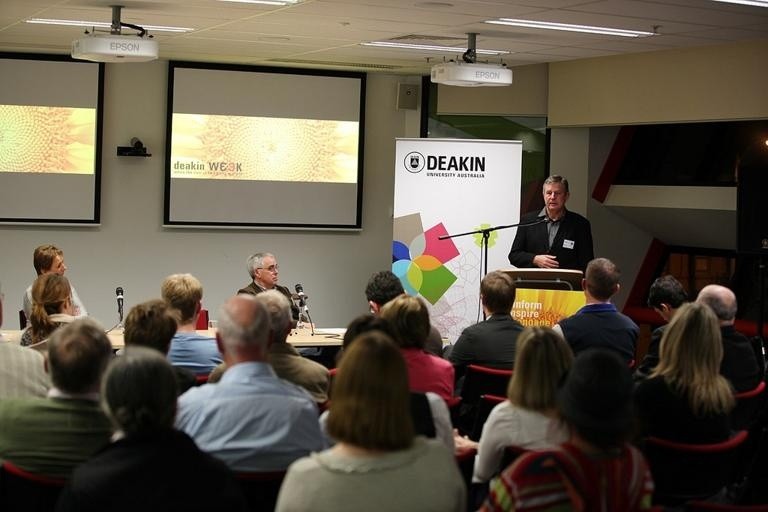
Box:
<box><xmin>0</xmin><ymin>459</ymin><xmax>78</xmax><ymax>512</ymax></box>
<box><xmin>464</xmin><ymin>347</ymin><xmax>768</xmax><ymax>511</ymax></box>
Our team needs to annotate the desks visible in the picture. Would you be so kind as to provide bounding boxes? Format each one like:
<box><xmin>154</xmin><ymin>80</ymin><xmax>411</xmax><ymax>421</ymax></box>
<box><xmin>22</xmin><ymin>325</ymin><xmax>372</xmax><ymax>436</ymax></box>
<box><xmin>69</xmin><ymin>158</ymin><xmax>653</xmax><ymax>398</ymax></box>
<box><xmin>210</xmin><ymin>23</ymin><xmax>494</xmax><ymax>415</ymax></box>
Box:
<box><xmin>0</xmin><ymin>328</ymin><xmax>357</xmax><ymax>358</ymax></box>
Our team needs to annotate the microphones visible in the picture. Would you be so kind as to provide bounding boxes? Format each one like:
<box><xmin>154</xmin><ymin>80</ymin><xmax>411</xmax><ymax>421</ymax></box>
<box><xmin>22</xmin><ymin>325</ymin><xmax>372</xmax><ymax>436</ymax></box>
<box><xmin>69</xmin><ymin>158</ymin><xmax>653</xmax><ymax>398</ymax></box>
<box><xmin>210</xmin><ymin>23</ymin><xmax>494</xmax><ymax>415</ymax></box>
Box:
<box><xmin>116</xmin><ymin>287</ymin><xmax>124</xmax><ymax>320</ymax></box>
<box><xmin>295</xmin><ymin>283</ymin><xmax>309</xmax><ymax>311</ymax></box>
<box><xmin>519</xmin><ymin>215</ymin><xmax>550</xmax><ymax>227</ymax></box>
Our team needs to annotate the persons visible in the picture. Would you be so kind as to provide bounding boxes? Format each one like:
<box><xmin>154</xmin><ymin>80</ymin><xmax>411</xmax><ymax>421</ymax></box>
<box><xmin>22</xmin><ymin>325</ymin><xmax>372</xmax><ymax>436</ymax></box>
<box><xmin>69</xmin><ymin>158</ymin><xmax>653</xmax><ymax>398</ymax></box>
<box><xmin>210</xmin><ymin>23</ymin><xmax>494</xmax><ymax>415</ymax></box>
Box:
<box><xmin>0</xmin><ymin>247</ymin><xmax>767</xmax><ymax>512</ymax></box>
<box><xmin>507</xmin><ymin>173</ymin><xmax>595</xmax><ymax>291</ymax></box>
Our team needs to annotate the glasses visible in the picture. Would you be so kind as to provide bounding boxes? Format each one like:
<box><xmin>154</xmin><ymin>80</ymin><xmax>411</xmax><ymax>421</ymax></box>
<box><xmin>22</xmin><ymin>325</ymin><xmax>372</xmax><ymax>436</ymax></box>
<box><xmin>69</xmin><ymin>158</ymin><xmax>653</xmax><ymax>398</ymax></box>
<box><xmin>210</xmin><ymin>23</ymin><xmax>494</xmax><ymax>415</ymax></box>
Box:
<box><xmin>257</xmin><ymin>265</ymin><xmax>278</xmax><ymax>272</ymax></box>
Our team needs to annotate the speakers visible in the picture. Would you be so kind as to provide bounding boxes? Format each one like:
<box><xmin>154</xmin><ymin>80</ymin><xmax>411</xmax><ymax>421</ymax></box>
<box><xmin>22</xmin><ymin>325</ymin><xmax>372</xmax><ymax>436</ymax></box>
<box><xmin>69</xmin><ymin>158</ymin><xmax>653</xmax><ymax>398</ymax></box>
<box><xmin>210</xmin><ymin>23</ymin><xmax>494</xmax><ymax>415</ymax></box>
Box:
<box><xmin>395</xmin><ymin>81</ymin><xmax>420</xmax><ymax>112</ymax></box>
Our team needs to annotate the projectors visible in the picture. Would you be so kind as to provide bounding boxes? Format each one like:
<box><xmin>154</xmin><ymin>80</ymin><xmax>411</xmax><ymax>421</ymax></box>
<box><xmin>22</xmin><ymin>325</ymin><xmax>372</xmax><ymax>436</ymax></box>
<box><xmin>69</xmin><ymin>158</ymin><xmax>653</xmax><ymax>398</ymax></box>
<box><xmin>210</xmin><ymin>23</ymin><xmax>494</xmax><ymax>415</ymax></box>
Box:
<box><xmin>430</xmin><ymin>32</ymin><xmax>513</xmax><ymax>87</ymax></box>
<box><xmin>71</xmin><ymin>4</ymin><xmax>158</xmax><ymax>64</ymax></box>
